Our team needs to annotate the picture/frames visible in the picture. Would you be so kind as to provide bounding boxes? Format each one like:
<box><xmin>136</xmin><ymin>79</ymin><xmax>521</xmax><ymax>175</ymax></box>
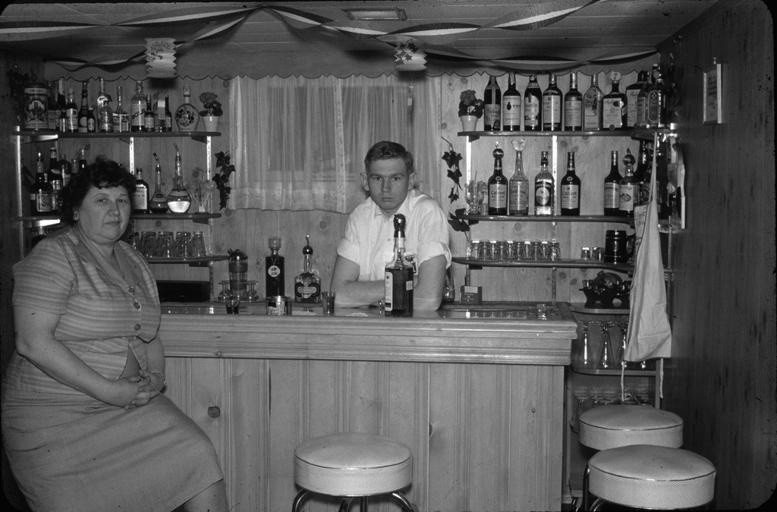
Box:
<box><xmin>702</xmin><ymin>64</ymin><xmax>723</xmax><ymax>123</ymax></box>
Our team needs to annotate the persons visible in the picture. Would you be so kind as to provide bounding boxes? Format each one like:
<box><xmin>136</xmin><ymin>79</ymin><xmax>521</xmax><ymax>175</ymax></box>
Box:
<box><xmin>0</xmin><ymin>158</ymin><xmax>228</xmax><ymax>512</ymax></box>
<box><xmin>328</xmin><ymin>141</ymin><xmax>453</xmax><ymax>312</ymax></box>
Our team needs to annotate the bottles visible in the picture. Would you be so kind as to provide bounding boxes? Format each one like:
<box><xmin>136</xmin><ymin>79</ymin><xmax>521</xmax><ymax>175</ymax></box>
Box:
<box><xmin>509</xmin><ymin>151</ymin><xmax>529</xmax><ymax>216</ymax></box>
<box><xmin>604</xmin><ymin>151</ymin><xmax>623</xmax><ymax>215</ymax></box>
<box><xmin>384</xmin><ymin>214</ymin><xmax>413</xmax><ymax>317</ymax></box>
<box><xmin>48</xmin><ymin>78</ymin><xmax>172</xmax><ymax>133</ymax></box>
<box><xmin>150</xmin><ymin>164</ymin><xmax>168</xmax><ymax>213</ymax></box>
<box><xmin>583</xmin><ymin>73</ymin><xmax>604</xmax><ymax>130</ymax></box>
<box><xmin>503</xmin><ymin>71</ymin><xmax>521</xmax><ymax>131</ymax></box>
<box><xmin>543</xmin><ymin>71</ymin><xmax>562</xmax><ymax>131</ymax></box>
<box><xmin>295</xmin><ymin>236</ymin><xmax>321</xmax><ymax>303</ymax></box>
<box><xmin>619</xmin><ymin>148</ymin><xmax>642</xmax><ymax>215</ymax></box>
<box><xmin>488</xmin><ymin>150</ymin><xmax>507</xmax><ymax>216</ymax></box>
<box><xmin>535</xmin><ymin>151</ymin><xmax>554</xmax><ymax>215</ymax></box>
<box><xmin>29</xmin><ymin>149</ymin><xmax>89</xmax><ymax>213</ymax></box>
<box><xmin>166</xmin><ymin>151</ymin><xmax>191</xmax><ymax>214</ymax></box>
<box><xmin>602</xmin><ymin>80</ymin><xmax>628</xmax><ymax>131</ymax></box>
<box><xmin>524</xmin><ymin>71</ymin><xmax>542</xmax><ymax>131</ymax></box>
<box><xmin>266</xmin><ymin>238</ymin><xmax>285</xmax><ymax>297</ymax></box>
<box><xmin>565</xmin><ymin>72</ymin><xmax>582</xmax><ymax>132</ymax></box>
<box><xmin>561</xmin><ymin>152</ymin><xmax>581</xmax><ymax>216</ymax></box>
<box><xmin>176</xmin><ymin>85</ymin><xmax>199</xmax><ymax>131</ymax></box>
<box><xmin>484</xmin><ymin>75</ymin><xmax>501</xmax><ymax>132</ymax></box>
<box><xmin>573</xmin><ymin>317</ymin><xmax>655</xmax><ymax>370</ymax></box>
<box><xmin>132</xmin><ymin>169</ymin><xmax>149</xmax><ymax>214</ymax></box>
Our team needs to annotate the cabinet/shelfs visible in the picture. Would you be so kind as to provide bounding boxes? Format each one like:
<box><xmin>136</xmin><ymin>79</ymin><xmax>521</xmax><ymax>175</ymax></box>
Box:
<box><xmin>452</xmin><ymin>126</ymin><xmax>641</xmax><ymax>276</ymax></box>
<box><xmin>567</xmin><ymin>302</ymin><xmax>666</xmax><ymax>499</ymax></box>
<box><xmin>12</xmin><ymin>124</ymin><xmax>230</xmax><ymax>265</ymax></box>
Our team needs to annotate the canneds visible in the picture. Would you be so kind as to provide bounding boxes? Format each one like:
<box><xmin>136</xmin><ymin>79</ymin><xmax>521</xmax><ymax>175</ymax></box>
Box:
<box><xmin>266</xmin><ymin>296</ymin><xmax>292</xmax><ymax>316</ymax></box>
<box><xmin>470</xmin><ymin>240</ymin><xmax>561</xmax><ymax>261</ymax></box>
<box><xmin>592</xmin><ymin>247</ymin><xmax>603</xmax><ymax>261</ymax></box>
<box><xmin>581</xmin><ymin>248</ymin><xmax>590</xmax><ymax>262</ymax></box>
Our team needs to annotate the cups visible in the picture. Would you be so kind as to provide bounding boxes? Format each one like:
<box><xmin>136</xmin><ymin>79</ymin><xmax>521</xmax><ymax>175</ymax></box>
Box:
<box><xmin>228</xmin><ymin>248</ymin><xmax>248</xmax><ymax>297</ymax></box>
<box><xmin>127</xmin><ymin>230</ymin><xmax>208</xmax><ymax>259</ymax></box>
<box><xmin>470</xmin><ymin>238</ymin><xmax>562</xmax><ymax>262</ymax></box>
<box><xmin>223</xmin><ymin>295</ymin><xmax>241</xmax><ymax>315</ymax></box>
<box><xmin>580</xmin><ymin>247</ymin><xmax>590</xmax><ymax>260</ymax></box>
<box><xmin>592</xmin><ymin>246</ymin><xmax>602</xmax><ymax>261</ymax></box>
<box><xmin>322</xmin><ymin>292</ymin><xmax>336</xmax><ymax>316</ymax></box>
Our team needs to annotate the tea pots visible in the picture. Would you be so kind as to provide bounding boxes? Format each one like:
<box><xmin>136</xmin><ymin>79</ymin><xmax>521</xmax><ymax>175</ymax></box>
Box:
<box><xmin>603</xmin><ymin>230</ymin><xmax>637</xmax><ymax>264</ymax></box>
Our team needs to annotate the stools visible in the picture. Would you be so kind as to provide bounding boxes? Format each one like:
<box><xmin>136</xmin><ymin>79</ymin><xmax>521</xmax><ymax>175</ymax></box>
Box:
<box><xmin>583</xmin><ymin>444</ymin><xmax>722</xmax><ymax>512</ymax></box>
<box><xmin>287</xmin><ymin>434</ymin><xmax>418</xmax><ymax>512</ymax></box>
<box><xmin>575</xmin><ymin>402</ymin><xmax>687</xmax><ymax>451</ymax></box>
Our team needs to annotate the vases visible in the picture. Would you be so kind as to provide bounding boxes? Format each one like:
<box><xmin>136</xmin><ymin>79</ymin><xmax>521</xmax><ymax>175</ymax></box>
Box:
<box><xmin>200</xmin><ymin>110</ymin><xmax>218</xmax><ymax>132</ymax></box>
<box><xmin>460</xmin><ymin>115</ymin><xmax>477</xmax><ymax>132</ymax></box>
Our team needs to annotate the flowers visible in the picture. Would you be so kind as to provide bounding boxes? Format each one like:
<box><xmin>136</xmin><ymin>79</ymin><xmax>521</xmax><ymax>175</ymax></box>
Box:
<box><xmin>196</xmin><ymin>90</ymin><xmax>222</xmax><ymax>117</ymax></box>
<box><xmin>457</xmin><ymin>90</ymin><xmax>483</xmax><ymax>116</ymax></box>
